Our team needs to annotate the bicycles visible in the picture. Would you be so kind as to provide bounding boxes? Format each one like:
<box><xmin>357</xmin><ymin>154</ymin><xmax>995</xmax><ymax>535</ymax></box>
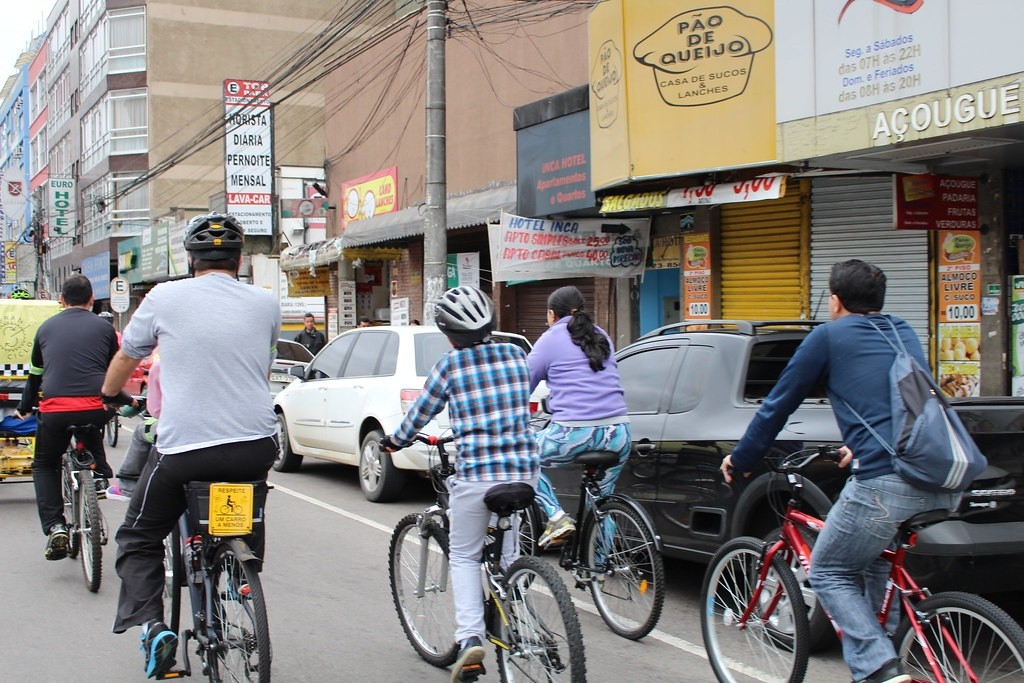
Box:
<box><xmin>16</xmin><ymin>403</ymin><xmax>110</xmax><ymax>593</ymax></box>
<box><xmin>700</xmin><ymin>443</ymin><xmax>1024</xmax><ymax>683</ymax></box>
<box><xmin>106</xmin><ymin>410</ymin><xmax>123</xmax><ymax>448</ymax></box>
<box><xmin>381</xmin><ymin>431</ymin><xmax>591</xmax><ymax>683</ymax></box>
<box><xmin>153</xmin><ymin>476</ymin><xmax>277</xmax><ymax>683</ymax></box>
<box><xmin>531</xmin><ymin>415</ymin><xmax>665</xmax><ymax>640</ymax></box>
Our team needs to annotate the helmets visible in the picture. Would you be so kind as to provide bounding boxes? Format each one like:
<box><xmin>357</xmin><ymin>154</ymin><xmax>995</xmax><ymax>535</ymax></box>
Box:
<box><xmin>11</xmin><ymin>289</ymin><xmax>30</xmax><ymax>299</ymax></box>
<box><xmin>435</xmin><ymin>286</ymin><xmax>496</xmax><ymax>345</ymax></box>
<box><xmin>184</xmin><ymin>211</ymin><xmax>245</xmax><ymax>259</ymax></box>
<box><xmin>98</xmin><ymin>311</ymin><xmax>114</xmax><ymax>321</ymax></box>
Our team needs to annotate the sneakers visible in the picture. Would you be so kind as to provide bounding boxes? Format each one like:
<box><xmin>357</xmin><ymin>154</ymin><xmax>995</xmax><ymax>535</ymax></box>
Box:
<box><xmin>572</xmin><ymin>570</ymin><xmax>605</xmax><ymax>595</ymax></box>
<box><xmin>538</xmin><ymin>513</ymin><xmax>576</xmax><ymax>547</ymax></box>
<box><xmin>95</xmin><ymin>477</ymin><xmax>110</xmax><ymax>499</ymax></box>
<box><xmin>140</xmin><ymin>619</ymin><xmax>178</xmax><ymax>679</ymax></box>
<box><xmin>227</xmin><ymin>577</ymin><xmax>252</xmax><ymax>603</ymax></box>
<box><xmin>45</xmin><ymin>522</ymin><xmax>69</xmax><ymax>560</ymax></box>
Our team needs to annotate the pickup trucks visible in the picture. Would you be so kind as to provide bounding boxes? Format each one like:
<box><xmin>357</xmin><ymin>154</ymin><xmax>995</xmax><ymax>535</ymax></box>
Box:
<box><xmin>529</xmin><ymin>317</ymin><xmax>1024</xmax><ymax>654</ymax></box>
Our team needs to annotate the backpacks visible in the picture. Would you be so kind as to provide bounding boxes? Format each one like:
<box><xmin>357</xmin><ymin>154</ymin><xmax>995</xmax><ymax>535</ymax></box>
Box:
<box><xmin>845</xmin><ymin>318</ymin><xmax>987</xmax><ymax>493</ymax></box>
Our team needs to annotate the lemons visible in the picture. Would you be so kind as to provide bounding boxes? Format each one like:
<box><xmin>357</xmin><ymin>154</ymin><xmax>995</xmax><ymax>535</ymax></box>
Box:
<box><xmin>938</xmin><ymin>337</ymin><xmax>981</xmax><ymax>361</ymax></box>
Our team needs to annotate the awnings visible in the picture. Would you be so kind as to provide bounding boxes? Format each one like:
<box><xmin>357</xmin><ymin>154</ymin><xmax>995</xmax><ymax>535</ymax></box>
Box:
<box><xmin>341</xmin><ymin>181</ymin><xmax>516</xmax><ymax>251</ymax></box>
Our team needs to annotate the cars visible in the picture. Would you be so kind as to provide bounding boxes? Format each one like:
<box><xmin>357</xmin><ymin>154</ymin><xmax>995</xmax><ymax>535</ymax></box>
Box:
<box><xmin>118</xmin><ymin>346</ymin><xmax>161</xmax><ymax>400</ymax></box>
<box><xmin>273</xmin><ymin>325</ymin><xmax>552</xmax><ymax>503</ymax></box>
<box><xmin>269</xmin><ymin>337</ymin><xmax>315</xmax><ymax>403</ymax></box>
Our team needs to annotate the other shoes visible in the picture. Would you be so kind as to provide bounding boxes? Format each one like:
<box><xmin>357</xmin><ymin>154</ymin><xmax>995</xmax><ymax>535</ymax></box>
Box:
<box><xmin>451</xmin><ymin>636</ymin><xmax>485</xmax><ymax>683</ymax></box>
<box><xmin>852</xmin><ymin>657</ymin><xmax>912</xmax><ymax>683</ymax></box>
<box><xmin>106</xmin><ymin>486</ymin><xmax>131</xmax><ymax>501</ymax></box>
<box><xmin>511</xmin><ymin>586</ymin><xmax>524</xmax><ymax>604</ymax></box>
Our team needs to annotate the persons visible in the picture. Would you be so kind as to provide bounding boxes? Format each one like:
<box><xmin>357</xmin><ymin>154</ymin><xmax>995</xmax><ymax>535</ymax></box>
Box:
<box><xmin>409</xmin><ymin>319</ymin><xmax>421</xmax><ymax>325</ymax></box>
<box><xmin>524</xmin><ymin>286</ymin><xmax>632</xmax><ymax>595</ymax></box>
<box><xmin>356</xmin><ymin>316</ymin><xmax>370</xmax><ymax>328</ymax></box>
<box><xmin>97</xmin><ymin>311</ymin><xmax>121</xmax><ymax>347</ymax></box>
<box><xmin>293</xmin><ymin>313</ymin><xmax>326</xmax><ymax>356</ymax></box>
<box><xmin>14</xmin><ymin>274</ymin><xmax>120</xmax><ymax>559</ymax></box>
<box><xmin>378</xmin><ymin>288</ymin><xmax>541</xmax><ymax>683</ymax></box>
<box><xmin>100</xmin><ymin>212</ymin><xmax>281</xmax><ymax>679</ymax></box>
<box><xmin>720</xmin><ymin>259</ymin><xmax>965</xmax><ymax>683</ymax></box>
<box><xmin>105</xmin><ymin>359</ymin><xmax>162</xmax><ymax>500</ymax></box>
